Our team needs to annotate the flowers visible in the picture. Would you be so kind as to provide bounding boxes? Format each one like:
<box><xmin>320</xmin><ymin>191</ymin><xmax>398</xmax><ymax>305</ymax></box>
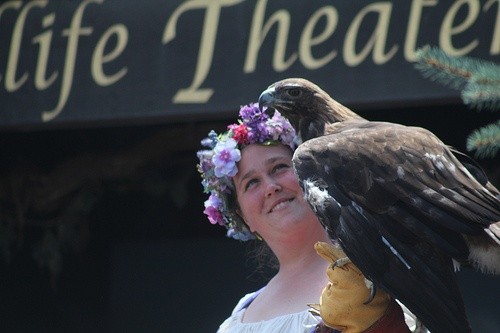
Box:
<box><xmin>196</xmin><ymin>101</ymin><xmax>303</xmax><ymax>243</ymax></box>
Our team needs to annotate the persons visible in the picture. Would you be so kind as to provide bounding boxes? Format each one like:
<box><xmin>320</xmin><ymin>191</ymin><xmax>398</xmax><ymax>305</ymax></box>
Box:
<box><xmin>196</xmin><ymin>101</ymin><xmax>431</xmax><ymax>333</ymax></box>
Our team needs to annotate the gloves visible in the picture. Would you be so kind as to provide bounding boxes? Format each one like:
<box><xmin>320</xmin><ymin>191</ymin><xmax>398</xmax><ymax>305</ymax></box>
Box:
<box><xmin>314</xmin><ymin>242</ymin><xmax>412</xmax><ymax>333</ymax></box>
